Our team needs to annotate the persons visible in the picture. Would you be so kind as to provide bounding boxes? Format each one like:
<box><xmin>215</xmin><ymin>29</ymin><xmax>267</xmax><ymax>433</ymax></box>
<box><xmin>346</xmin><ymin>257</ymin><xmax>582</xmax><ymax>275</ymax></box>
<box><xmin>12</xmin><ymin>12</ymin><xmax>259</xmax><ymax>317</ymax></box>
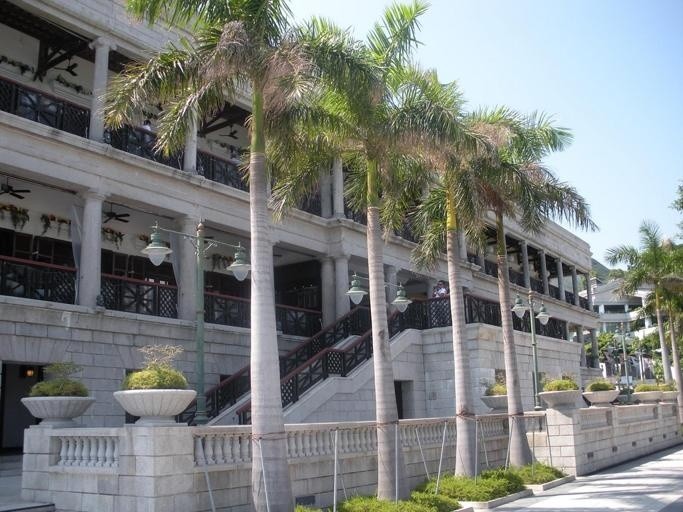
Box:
<box><xmin>134</xmin><ymin>119</ymin><xmax>152</xmax><ymax>157</ymax></box>
<box><xmin>430</xmin><ymin>279</ymin><xmax>452</xmax><ymax>326</ymax></box>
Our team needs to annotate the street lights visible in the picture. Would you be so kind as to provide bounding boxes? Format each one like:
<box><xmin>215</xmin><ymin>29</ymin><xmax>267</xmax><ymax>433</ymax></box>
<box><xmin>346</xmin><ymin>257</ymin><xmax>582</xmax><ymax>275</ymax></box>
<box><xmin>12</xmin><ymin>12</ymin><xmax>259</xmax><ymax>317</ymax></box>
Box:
<box><xmin>347</xmin><ymin>274</ymin><xmax>411</xmax><ymax>313</ymax></box>
<box><xmin>511</xmin><ymin>288</ymin><xmax>551</xmax><ymax>411</ymax></box>
<box><xmin>143</xmin><ymin>222</ymin><xmax>249</xmax><ymax>427</ymax></box>
<box><xmin>615</xmin><ymin>326</ymin><xmax>632</xmax><ymax>389</ymax></box>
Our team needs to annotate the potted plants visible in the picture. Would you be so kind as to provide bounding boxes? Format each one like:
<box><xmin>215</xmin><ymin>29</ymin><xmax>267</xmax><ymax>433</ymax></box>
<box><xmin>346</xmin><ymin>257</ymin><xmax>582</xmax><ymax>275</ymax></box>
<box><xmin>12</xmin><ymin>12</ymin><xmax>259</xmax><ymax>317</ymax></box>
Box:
<box><xmin>479</xmin><ymin>384</ymin><xmax>509</xmax><ymax>414</ymax></box>
<box><xmin>115</xmin><ymin>343</ymin><xmax>197</xmax><ymax>423</ymax></box>
<box><xmin>18</xmin><ymin>363</ymin><xmax>96</xmax><ymax>430</ymax></box>
<box><xmin>537</xmin><ymin>375</ymin><xmax>679</xmax><ymax>409</ymax></box>
